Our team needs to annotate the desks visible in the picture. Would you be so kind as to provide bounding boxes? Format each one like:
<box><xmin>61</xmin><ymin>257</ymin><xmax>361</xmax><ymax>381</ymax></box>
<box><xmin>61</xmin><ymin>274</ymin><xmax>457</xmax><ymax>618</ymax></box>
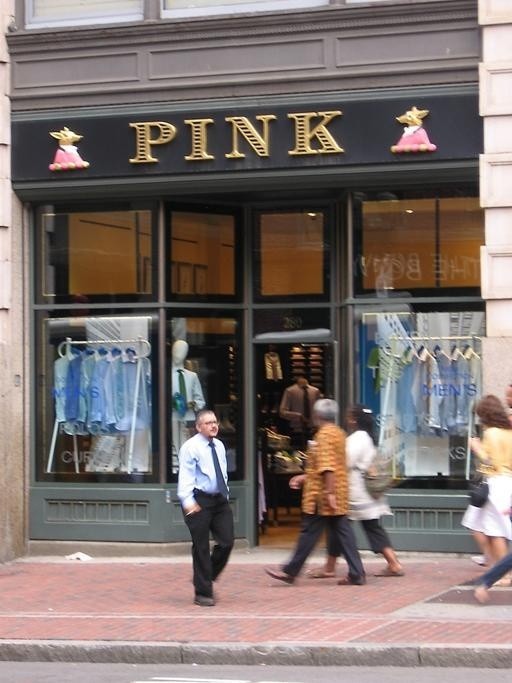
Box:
<box><xmin>268</xmin><ymin>471</ymin><xmax>305</xmax><ymax>527</ymax></box>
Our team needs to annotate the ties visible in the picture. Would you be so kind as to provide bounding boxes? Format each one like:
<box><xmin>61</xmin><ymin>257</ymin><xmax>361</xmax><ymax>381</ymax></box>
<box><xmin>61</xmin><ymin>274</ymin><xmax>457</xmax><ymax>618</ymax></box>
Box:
<box><xmin>177</xmin><ymin>369</ymin><xmax>186</xmax><ymax>402</ymax></box>
<box><xmin>301</xmin><ymin>386</ymin><xmax>311</xmax><ymax>417</ymax></box>
<box><xmin>209</xmin><ymin>443</ymin><xmax>229</xmax><ymax>498</ymax></box>
<box><xmin>269</xmin><ymin>358</ymin><xmax>278</xmax><ymax>382</ymax></box>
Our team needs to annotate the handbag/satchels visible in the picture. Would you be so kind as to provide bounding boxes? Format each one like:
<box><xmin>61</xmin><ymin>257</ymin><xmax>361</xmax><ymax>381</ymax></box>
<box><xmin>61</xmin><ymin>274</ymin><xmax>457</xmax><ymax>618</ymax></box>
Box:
<box><xmin>365</xmin><ymin>467</ymin><xmax>391</xmax><ymax>497</ymax></box>
<box><xmin>468</xmin><ymin>481</ymin><xmax>488</xmax><ymax>507</ymax></box>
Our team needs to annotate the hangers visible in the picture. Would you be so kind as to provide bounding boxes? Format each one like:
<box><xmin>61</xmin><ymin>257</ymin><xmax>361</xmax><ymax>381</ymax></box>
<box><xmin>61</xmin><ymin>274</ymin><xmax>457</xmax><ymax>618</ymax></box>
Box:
<box><xmin>397</xmin><ymin>335</ymin><xmax>480</xmax><ymax>360</ymax></box>
<box><xmin>63</xmin><ymin>340</ymin><xmax>137</xmax><ymax>361</ymax></box>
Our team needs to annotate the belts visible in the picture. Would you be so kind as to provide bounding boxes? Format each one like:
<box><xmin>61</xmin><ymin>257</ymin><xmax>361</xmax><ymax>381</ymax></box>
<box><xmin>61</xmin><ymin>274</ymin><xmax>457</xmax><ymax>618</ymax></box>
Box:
<box><xmin>195</xmin><ymin>490</ymin><xmax>219</xmax><ymax>500</ymax></box>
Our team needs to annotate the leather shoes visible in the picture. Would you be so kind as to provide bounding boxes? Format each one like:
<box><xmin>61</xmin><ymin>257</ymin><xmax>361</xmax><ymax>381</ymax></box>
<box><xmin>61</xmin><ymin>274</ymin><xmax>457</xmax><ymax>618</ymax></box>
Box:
<box><xmin>194</xmin><ymin>596</ymin><xmax>215</xmax><ymax>606</ymax></box>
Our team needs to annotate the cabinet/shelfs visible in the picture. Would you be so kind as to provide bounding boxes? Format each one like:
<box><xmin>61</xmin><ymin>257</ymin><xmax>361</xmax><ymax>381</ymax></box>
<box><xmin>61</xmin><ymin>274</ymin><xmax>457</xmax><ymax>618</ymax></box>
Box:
<box><xmin>290</xmin><ymin>350</ymin><xmax>324</xmax><ymax>391</ymax></box>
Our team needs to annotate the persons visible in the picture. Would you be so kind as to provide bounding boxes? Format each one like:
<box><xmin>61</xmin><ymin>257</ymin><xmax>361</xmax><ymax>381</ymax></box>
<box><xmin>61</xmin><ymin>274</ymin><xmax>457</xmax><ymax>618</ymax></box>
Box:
<box><xmin>279</xmin><ymin>376</ymin><xmax>321</xmax><ymax>448</ymax></box>
<box><xmin>505</xmin><ymin>384</ymin><xmax>511</xmax><ymax>421</ymax></box>
<box><xmin>170</xmin><ymin>340</ymin><xmax>204</xmax><ymax>477</ymax></box>
<box><xmin>460</xmin><ymin>552</ymin><xmax>512</xmax><ymax>604</ymax></box>
<box><xmin>264</xmin><ymin>398</ymin><xmax>366</xmax><ymax>585</ymax></box>
<box><xmin>304</xmin><ymin>404</ymin><xmax>407</xmax><ymax>579</ymax></box>
<box><xmin>461</xmin><ymin>394</ymin><xmax>512</xmax><ymax>585</ymax></box>
<box><xmin>178</xmin><ymin>409</ymin><xmax>234</xmax><ymax>605</ymax></box>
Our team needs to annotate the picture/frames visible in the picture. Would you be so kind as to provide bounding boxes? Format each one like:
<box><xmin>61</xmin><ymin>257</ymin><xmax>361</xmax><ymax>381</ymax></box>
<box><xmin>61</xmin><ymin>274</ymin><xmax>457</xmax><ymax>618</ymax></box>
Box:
<box><xmin>144</xmin><ymin>256</ymin><xmax>208</xmax><ymax>294</ymax></box>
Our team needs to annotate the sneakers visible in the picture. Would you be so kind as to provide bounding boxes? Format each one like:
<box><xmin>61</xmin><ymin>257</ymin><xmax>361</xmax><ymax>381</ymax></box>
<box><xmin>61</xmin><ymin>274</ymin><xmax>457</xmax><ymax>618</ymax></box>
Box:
<box><xmin>265</xmin><ymin>567</ymin><xmax>293</xmax><ymax>585</ymax></box>
<box><xmin>309</xmin><ymin>568</ymin><xmax>335</xmax><ymax>578</ymax></box>
<box><xmin>375</xmin><ymin>566</ymin><xmax>405</xmax><ymax>576</ymax></box>
<box><xmin>473</xmin><ymin>554</ymin><xmax>510</xmax><ymax>603</ymax></box>
<box><xmin>336</xmin><ymin>579</ymin><xmax>357</xmax><ymax>583</ymax></box>
